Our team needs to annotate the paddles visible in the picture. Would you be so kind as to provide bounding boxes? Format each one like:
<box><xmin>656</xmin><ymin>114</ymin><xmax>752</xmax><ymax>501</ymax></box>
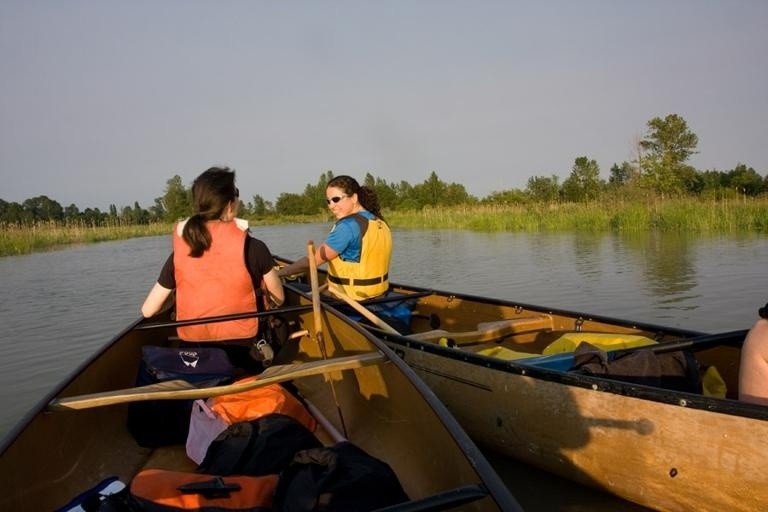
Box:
<box><xmin>306</xmin><ymin>239</ymin><xmax>349</xmax><ymax>439</ymax></box>
<box><xmin>256</xmin><ymin>341</ymin><xmax>410</xmax><ymax>510</ymax></box>
<box><xmin>509</xmin><ymin>328</ymin><xmax>747</xmax><ymax>376</ymax></box>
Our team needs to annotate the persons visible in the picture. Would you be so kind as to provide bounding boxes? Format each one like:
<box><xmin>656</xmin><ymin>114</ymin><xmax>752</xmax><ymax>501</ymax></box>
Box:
<box><xmin>276</xmin><ymin>176</ymin><xmax>391</xmax><ymax>302</ymax></box>
<box><xmin>139</xmin><ymin>168</ymin><xmax>285</xmax><ymax>340</ymax></box>
<box><xmin>737</xmin><ymin>302</ymin><xmax>768</xmax><ymax>408</ymax></box>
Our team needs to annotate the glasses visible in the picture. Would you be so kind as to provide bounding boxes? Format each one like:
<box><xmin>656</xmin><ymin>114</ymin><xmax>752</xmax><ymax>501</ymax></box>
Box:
<box><xmin>325</xmin><ymin>195</ymin><xmax>349</xmax><ymax>204</ymax></box>
